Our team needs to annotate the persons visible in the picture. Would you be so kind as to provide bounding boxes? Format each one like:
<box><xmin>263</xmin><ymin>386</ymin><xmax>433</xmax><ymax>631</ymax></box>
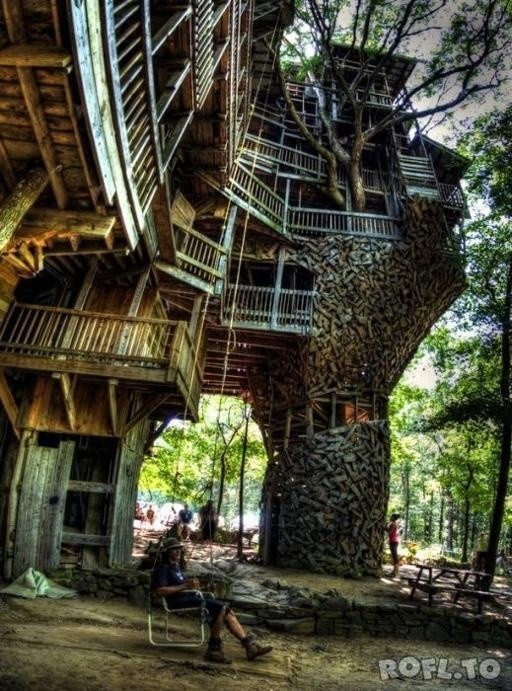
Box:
<box><xmin>178</xmin><ymin>505</ymin><xmax>193</xmax><ymax>541</ymax></box>
<box><xmin>384</xmin><ymin>512</ymin><xmax>402</xmax><ymax>577</ymax></box>
<box><xmin>150</xmin><ymin>536</ymin><xmax>273</xmax><ymax>664</ymax></box>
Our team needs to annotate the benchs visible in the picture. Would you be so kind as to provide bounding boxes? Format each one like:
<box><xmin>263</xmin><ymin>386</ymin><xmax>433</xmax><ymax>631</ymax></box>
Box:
<box><xmin>399</xmin><ymin>575</ymin><xmax>500</xmax><ymax>614</ymax></box>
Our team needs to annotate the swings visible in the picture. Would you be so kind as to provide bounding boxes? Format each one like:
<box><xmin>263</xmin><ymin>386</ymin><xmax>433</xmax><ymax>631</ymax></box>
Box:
<box><xmin>145</xmin><ymin>2</ymin><xmax>290</xmax><ymax>648</ymax></box>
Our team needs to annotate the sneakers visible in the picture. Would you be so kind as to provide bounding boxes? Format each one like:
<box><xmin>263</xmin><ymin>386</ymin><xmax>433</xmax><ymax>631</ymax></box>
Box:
<box><xmin>247</xmin><ymin>644</ymin><xmax>272</xmax><ymax>658</ymax></box>
<box><xmin>205</xmin><ymin>649</ymin><xmax>232</xmax><ymax>663</ymax></box>
<box><xmin>386</xmin><ymin>571</ymin><xmax>395</xmax><ymax>577</ymax></box>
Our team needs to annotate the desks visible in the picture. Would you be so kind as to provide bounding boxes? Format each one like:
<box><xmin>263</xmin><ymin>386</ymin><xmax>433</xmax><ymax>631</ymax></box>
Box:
<box><xmin>408</xmin><ymin>565</ymin><xmax>492</xmax><ymax>614</ymax></box>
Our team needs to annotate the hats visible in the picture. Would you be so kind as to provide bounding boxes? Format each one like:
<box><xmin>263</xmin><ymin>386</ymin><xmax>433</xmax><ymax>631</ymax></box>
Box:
<box><xmin>162</xmin><ymin>538</ymin><xmax>183</xmax><ymax>552</ymax></box>
